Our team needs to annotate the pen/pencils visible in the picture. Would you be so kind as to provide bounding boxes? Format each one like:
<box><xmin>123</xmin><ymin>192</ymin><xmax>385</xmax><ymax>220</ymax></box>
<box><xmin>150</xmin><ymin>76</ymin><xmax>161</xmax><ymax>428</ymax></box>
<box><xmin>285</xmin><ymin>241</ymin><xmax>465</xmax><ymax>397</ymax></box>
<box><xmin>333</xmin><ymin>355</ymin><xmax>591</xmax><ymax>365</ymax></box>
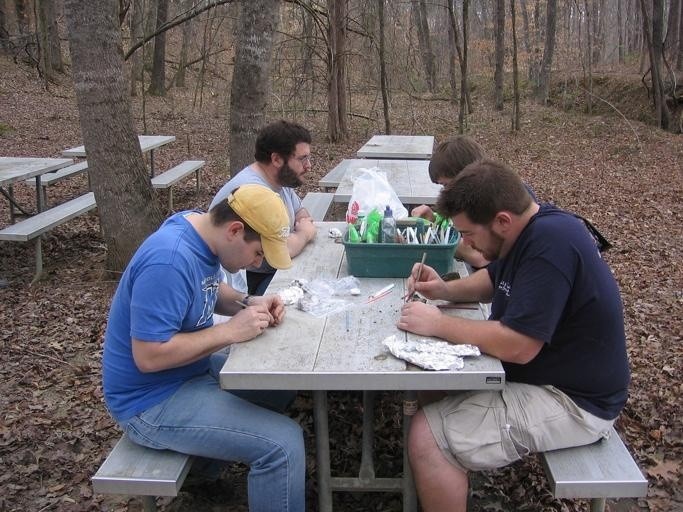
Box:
<box><xmin>396</xmin><ymin>224</ymin><xmax>451</xmax><ymax>244</ymax></box>
<box><xmin>412</xmin><ymin>252</ymin><xmax>427</xmax><ymax>298</ymax></box>
<box><xmin>437</xmin><ymin>305</ymin><xmax>479</xmax><ymax>310</ymax></box>
<box><xmin>236</xmin><ymin>300</ymin><xmax>248</xmax><ymax>307</ymax></box>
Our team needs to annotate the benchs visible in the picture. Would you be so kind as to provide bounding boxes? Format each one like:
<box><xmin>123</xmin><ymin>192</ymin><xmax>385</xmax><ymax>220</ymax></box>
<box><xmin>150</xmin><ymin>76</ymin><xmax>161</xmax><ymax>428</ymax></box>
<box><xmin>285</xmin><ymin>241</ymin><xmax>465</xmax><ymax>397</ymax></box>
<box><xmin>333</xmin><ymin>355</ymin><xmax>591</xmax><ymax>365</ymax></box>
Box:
<box><xmin>151</xmin><ymin>161</ymin><xmax>207</xmax><ymax>216</ymax></box>
<box><xmin>26</xmin><ymin>160</ymin><xmax>91</xmax><ymax>211</ymax></box>
<box><xmin>539</xmin><ymin>426</ymin><xmax>648</xmax><ymax>512</ymax></box>
<box><xmin>89</xmin><ymin>434</ymin><xmax>191</xmax><ymax>512</ymax></box>
<box><xmin>0</xmin><ymin>192</ymin><xmax>98</xmax><ymax>286</ymax></box>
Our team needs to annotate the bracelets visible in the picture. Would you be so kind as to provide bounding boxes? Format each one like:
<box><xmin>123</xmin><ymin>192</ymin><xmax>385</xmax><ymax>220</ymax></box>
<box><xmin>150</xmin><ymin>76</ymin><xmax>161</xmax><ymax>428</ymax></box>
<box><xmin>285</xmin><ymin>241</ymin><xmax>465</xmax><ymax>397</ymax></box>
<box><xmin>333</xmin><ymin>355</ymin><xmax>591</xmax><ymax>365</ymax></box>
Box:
<box><xmin>242</xmin><ymin>295</ymin><xmax>251</xmax><ymax>309</ymax></box>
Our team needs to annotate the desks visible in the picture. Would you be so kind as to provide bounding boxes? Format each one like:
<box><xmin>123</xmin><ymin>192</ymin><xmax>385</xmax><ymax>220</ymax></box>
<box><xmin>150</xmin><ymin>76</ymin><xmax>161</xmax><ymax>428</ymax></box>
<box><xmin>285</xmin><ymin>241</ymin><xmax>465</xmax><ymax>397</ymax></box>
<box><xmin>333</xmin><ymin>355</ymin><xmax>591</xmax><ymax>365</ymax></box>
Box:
<box><xmin>61</xmin><ymin>135</ymin><xmax>175</xmax><ymax>194</ymax></box>
<box><xmin>0</xmin><ymin>156</ymin><xmax>73</xmax><ymax>224</ymax></box>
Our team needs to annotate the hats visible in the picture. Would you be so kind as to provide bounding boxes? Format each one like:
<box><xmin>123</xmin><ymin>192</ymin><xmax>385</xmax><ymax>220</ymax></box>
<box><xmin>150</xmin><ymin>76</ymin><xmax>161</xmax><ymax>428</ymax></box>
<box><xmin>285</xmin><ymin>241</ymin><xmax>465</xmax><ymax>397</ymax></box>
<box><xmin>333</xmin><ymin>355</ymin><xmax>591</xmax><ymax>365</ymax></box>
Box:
<box><xmin>227</xmin><ymin>183</ymin><xmax>292</xmax><ymax>270</ymax></box>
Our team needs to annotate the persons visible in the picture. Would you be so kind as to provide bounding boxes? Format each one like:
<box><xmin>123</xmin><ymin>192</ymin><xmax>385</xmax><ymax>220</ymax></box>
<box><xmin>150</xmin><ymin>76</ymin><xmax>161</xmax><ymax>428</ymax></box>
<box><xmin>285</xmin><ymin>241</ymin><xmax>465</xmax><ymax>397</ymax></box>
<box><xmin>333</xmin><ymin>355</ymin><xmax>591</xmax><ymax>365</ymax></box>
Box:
<box><xmin>410</xmin><ymin>135</ymin><xmax>535</xmax><ymax>270</ymax></box>
<box><xmin>208</xmin><ymin>121</ymin><xmax>316</xmax><ymax>295</ymax></box>
<box><xmin>398</xmin><ymin>161</ymin><xmax>627</xmax><ymax>512</ymax></box>
<box><xmin>102</xmin><ymin>182</ymin><xmax>306</xmax><ymax>512</ymax></box>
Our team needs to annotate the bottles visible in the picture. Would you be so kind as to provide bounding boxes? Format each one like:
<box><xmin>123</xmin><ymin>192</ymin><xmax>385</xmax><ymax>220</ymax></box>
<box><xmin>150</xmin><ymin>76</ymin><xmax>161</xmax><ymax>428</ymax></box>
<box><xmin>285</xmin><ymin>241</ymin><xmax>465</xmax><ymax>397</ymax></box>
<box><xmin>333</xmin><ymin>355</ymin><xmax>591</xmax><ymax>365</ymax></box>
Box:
<box><xmin>380</xmin><ymin>206</ymin><xmax>397</xmax><ymax>244</ymax></box>
<box><xmin>413</xmin><ymin>219</ymin><xmax>425</xmax><ymax>241</ymax></box>
<box><xmin>355</xmin><ymin>210</ymin><xmax>365</xmax><ymax>225</ymax></box>
